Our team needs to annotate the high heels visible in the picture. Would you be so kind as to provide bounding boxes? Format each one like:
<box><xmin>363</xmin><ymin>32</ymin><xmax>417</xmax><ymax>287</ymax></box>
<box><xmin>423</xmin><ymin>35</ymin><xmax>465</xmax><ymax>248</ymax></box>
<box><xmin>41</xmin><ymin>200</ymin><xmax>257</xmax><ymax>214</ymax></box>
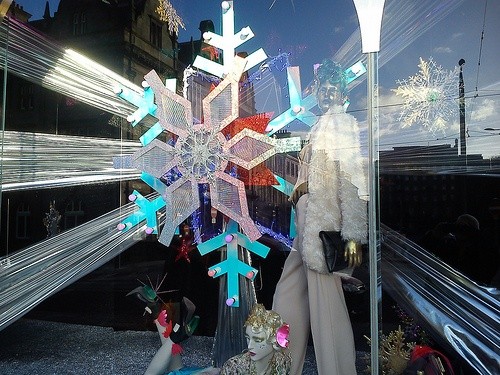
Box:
<box><xmin>126</xmin><ymin>286</ymin><xmax>161</xmax><ymax>323</ymax></box>
<box><xmin>170</xmin><ymin>296</ymin><xmax>201</xmax><ymax>344</ymax></box>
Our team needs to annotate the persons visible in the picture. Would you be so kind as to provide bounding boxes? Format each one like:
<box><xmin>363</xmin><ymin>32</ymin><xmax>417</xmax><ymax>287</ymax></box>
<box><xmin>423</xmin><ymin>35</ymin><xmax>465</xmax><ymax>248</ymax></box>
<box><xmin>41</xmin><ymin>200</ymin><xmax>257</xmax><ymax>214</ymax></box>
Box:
<box><xmin>142</xmin><ymin>296</ymin><xmax>294</xmax><ymax>375</ymax></box>
<box><xmin>263</xmin><ymin>60</ymin><xmax>371</xmax><ymax>375</ymax></box>
<box><xmin>415</xmin><ymin>212</ymin><xmax>500</xmax><ymax>286</ymax></box>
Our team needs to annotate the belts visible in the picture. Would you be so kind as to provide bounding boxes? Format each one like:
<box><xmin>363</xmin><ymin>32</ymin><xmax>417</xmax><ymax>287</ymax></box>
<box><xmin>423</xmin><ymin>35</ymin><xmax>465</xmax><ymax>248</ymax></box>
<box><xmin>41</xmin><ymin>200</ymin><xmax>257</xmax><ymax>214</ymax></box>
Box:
<box><xmin>291</xmin><ymin>181</ymin><xmax>309</xmax><ymax>208</ymax></box>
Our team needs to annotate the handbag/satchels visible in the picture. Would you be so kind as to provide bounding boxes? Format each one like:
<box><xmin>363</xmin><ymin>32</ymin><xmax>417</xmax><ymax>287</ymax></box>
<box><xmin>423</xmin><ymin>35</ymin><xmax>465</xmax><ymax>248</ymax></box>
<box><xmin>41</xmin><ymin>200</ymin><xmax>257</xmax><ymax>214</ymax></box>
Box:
<box><xmin>319</xmin><ymin>231</ymin><xmax>355</xmax><ymax>272</ymax></box>
